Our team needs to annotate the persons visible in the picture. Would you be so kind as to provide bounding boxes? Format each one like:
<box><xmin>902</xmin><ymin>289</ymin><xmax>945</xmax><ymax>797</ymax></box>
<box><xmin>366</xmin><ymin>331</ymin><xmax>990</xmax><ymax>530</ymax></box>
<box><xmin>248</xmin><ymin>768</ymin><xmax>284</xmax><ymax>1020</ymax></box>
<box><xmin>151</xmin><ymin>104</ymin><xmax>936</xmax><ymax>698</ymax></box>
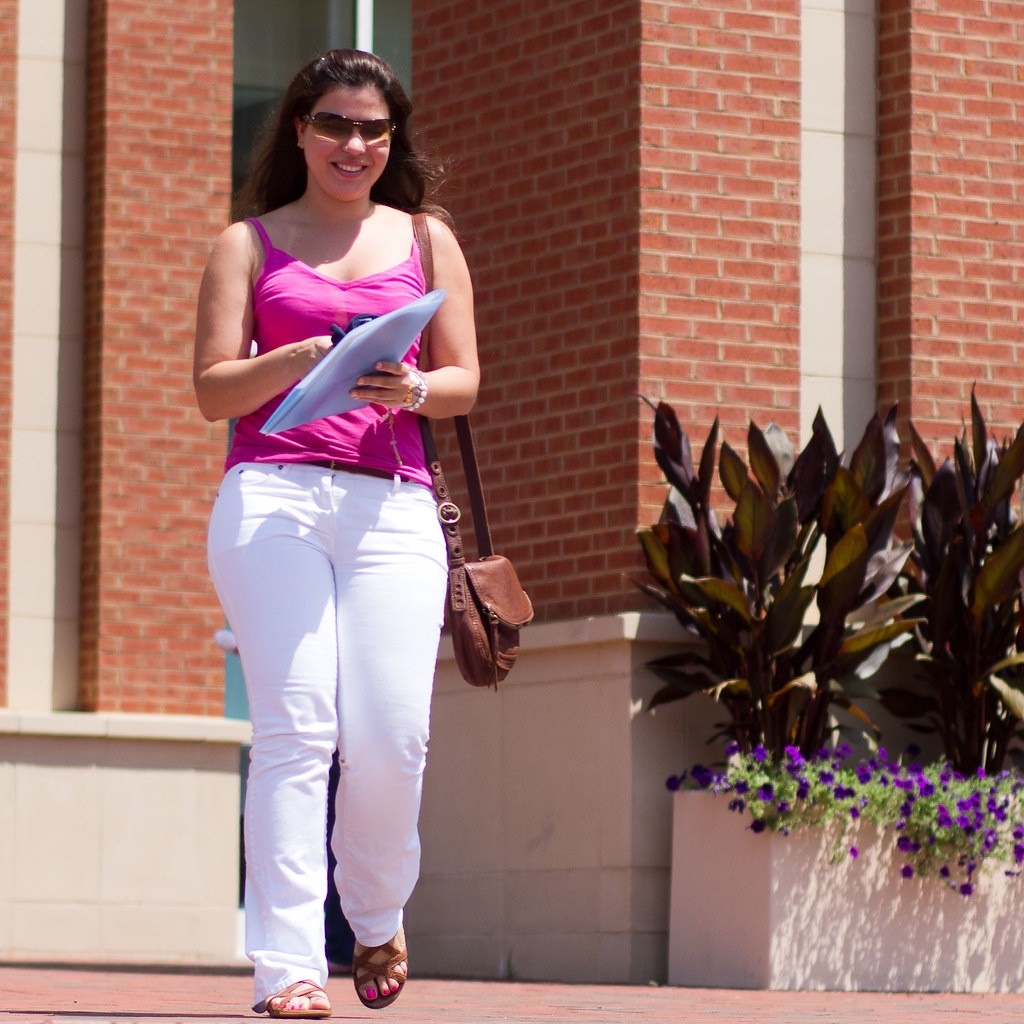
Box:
<box><xmin>194</xmin><ymin>47</ymin><xmax>481</xmax><ymax>1017</ymax></box>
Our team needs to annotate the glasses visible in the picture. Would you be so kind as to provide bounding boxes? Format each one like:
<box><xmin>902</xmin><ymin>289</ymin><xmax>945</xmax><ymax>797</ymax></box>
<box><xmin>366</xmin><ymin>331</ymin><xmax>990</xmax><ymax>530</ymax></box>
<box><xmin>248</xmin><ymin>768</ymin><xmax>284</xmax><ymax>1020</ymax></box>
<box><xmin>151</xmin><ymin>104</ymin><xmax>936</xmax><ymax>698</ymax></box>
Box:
<box><xmin>301</xmin><ymin>112</ymin><xmax>398</xmax><ymax>144</ymax></box>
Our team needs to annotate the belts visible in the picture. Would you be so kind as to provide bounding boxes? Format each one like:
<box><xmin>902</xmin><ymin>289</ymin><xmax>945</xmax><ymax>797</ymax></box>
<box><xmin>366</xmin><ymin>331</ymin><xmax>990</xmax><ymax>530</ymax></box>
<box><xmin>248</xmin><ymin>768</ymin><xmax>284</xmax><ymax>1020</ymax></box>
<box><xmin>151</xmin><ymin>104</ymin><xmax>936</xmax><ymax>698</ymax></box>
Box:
<box><xmin>303</xmin><ymin>459</ymin><xmax>410</xmax><ymax>483</ymax></box>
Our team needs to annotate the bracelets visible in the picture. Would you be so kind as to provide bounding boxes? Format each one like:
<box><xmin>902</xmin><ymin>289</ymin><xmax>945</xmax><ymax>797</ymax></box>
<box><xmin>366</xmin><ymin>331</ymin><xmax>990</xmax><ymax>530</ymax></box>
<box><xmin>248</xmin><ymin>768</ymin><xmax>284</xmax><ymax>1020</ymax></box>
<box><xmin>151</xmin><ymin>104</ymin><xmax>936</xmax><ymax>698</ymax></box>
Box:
<box><xmin>401</xmin><ymin>373</ymin><xmax>427</xmax><ymax>411</ymax></box>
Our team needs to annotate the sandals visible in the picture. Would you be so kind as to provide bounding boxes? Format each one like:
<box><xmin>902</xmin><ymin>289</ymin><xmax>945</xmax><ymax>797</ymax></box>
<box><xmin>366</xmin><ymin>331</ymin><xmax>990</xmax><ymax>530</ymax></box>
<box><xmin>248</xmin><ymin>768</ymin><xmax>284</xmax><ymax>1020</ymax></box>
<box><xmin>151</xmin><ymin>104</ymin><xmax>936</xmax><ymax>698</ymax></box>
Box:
<box><xmin>266</xmin><ymin>980</ymin><xmax>333</xmax><ymax>1017</ymax></box>
<box><xmin>352</xmin><ymin>927</ymin><xmax>408</xmax><ymax>1009</ymax></box>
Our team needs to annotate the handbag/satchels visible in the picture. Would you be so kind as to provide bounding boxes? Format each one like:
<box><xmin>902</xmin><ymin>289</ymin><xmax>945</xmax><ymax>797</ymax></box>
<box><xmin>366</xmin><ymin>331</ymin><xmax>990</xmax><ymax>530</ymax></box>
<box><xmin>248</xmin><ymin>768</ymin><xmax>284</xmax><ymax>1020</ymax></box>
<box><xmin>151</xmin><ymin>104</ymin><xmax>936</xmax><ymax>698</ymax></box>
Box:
<box><xmin>448</xmin><ymin>554</ymin><xmax>535</xmax><ymax>694</ymax></box>
